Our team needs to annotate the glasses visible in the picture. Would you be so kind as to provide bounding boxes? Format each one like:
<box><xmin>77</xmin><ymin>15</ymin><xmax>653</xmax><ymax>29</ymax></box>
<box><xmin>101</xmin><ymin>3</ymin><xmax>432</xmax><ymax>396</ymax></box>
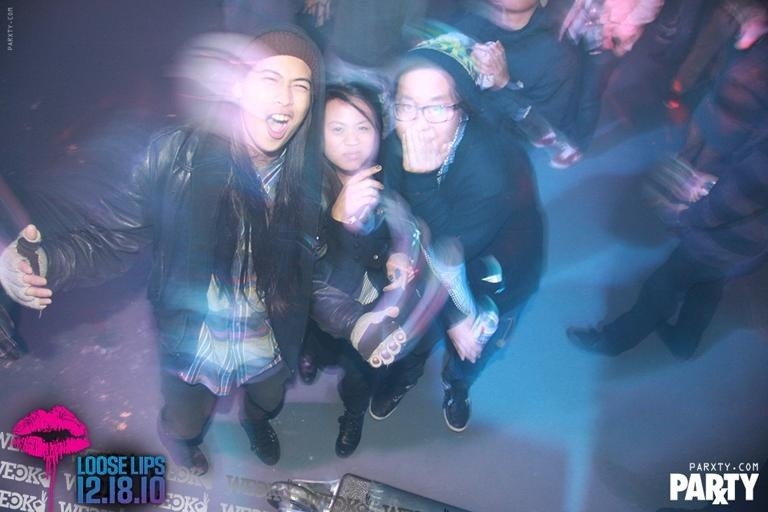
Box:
<box><xmin>391</xmin><ymin>102</ymin><xmax>462</xmax><ymax>123</ymax></box>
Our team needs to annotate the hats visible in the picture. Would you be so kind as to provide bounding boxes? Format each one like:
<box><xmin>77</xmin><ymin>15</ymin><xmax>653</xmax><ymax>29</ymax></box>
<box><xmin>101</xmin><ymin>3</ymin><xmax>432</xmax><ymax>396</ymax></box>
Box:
<box><xmin>405</xmin><ymin>31</ymin><xmax>478</xmax><ymax>101</ymax></box>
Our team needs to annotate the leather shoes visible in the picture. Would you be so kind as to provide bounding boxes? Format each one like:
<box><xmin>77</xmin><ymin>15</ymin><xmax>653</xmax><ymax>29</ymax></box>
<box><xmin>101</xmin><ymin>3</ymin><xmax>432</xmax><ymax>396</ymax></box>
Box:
<box><xmin>335</xmin><ymin>410</ymin><xmax>363</xmax><ymax>457</ymax></box>
<box><xmin>299</xmin><ymin>356</ymin><xmax>317</xmax><ymax>384</ymax></box>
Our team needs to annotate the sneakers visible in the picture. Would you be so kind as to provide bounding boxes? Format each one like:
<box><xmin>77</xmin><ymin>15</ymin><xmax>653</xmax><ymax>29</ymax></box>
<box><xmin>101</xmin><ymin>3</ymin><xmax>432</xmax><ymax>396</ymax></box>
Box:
<box><xmin>566</xmin><ymin>326</ymin><xmax>623</xmax><ymax>356</ymax></box>
<box><xmin>550</xmin><ymin>145</ymin><xmax>582</xmax><ymax>169</ymax></box>
<box><xmin>441</xmin><ymin>377</ymin><xmax>470</xmax><ymax>432</ymax></box>
<box><xmin>238</xmin><ymin>410</ymin><xmax>279</xmax><ymax>466</ymax></box>
<box><xmin>156</xmin><ymin>432</ymin><xmax>206</xmax><ymax>477</ymax></box>
<box><xmin>369</xmin><ymin>379</ymin><xmax>414</xmax><ymax>419</ymax></box>
<box><xmin>532</xmin><ymin>129</ymin><xmax>558</xmax><ymax>148</ymax></box>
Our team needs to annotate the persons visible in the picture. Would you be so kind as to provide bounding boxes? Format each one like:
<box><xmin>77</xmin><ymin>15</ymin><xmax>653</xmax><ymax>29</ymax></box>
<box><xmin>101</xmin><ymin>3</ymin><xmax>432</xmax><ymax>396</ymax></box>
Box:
<box><xmin>1</xmin><ymin>17</ymin><xmax>323</xmax><ymax>477</ymax></box>
<box><xmin>272</xmin><ymin>0</ymin><xmax>763</xmax><ymax>360</ymax></box>
<box><xmin>287</xmin><ymin>78</ymin><xmax>422</xmax><ymax>457</ymax></box>
<box><xmin>365</xmin><ymin>48</ymin><xmax>544</xmax><ymax>432</ymax></box>
